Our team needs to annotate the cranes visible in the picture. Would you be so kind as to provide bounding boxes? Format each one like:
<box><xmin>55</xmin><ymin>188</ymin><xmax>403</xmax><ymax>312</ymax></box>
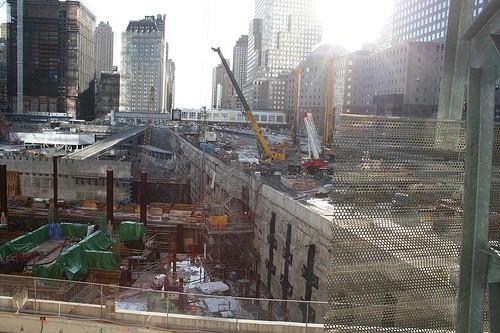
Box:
<box><xmin>322</xmin><ymin>55</ymin><xmax>337</xmax><ymax>163</ymax></box>
<box><xmin>211</xmin><ymin>46</ymin><xmax>286</xmax><ymax>175</ymax></box>
<box><xmin>282</xmin><ymin>64</ymin><xmax>303</xmax><ymax>155</ymax></box>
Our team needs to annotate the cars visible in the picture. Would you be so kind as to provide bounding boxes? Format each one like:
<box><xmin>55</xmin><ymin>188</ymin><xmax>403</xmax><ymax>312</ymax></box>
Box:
<box><xmin>391</xmin><ymin>192</ymin><xmax>410</xmax><ymax>206</ymax></box>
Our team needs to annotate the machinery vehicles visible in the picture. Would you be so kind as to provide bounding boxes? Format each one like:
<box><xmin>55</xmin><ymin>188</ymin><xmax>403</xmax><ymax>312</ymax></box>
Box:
<box><xmin>288</xmin><ymin>159</ymin><xmax>330</xmax><ymax>179</ymax></box>
